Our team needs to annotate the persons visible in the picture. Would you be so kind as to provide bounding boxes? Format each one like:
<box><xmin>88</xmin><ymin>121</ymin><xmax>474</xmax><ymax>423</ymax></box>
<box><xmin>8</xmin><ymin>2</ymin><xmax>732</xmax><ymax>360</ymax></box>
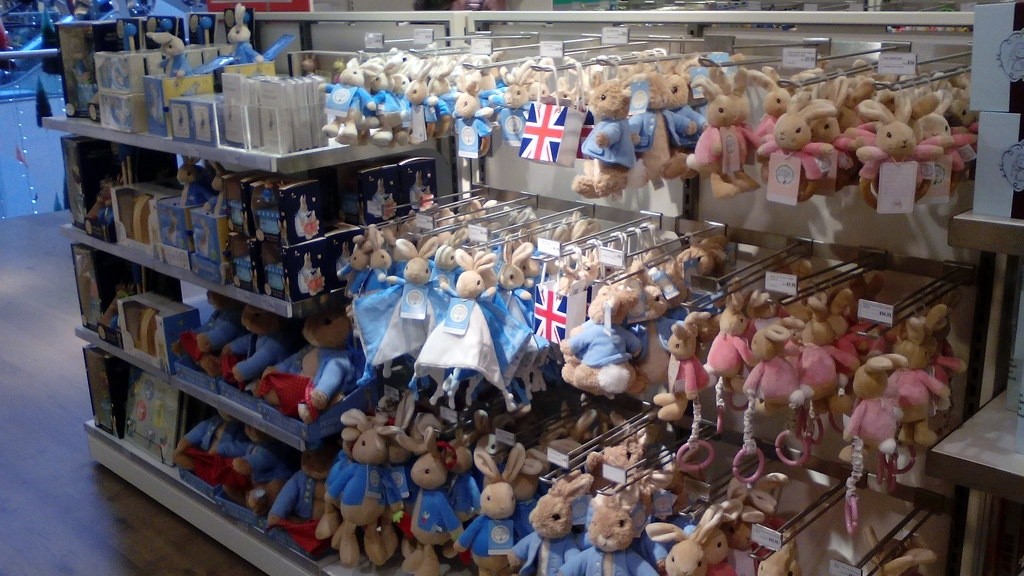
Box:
<box><xmin>0</xmin><ymin>6</ymin><xmax>16</xmax><ymax>70</ymax></box>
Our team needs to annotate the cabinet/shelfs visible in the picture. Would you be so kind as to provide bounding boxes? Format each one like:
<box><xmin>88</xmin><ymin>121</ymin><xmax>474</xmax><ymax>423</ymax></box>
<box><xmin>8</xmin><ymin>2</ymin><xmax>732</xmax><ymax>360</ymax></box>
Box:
<box><xmin>39</xmin><ymin>8</ymin><xmax>470</xmax><ymax>576</ymax></box>
<box><xmin>929</xmin><ymin>215</ymin><xmax>1024</xmax><ymax>504</ymax></box>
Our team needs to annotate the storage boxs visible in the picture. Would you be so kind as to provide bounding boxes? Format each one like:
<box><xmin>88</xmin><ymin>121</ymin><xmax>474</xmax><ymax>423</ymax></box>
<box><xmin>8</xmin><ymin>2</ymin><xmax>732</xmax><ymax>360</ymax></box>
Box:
<box><xmin>967</xmin><ymin>2</ymin><xmax>1024</xmax><ymax>220</ymax></box>
<box><xmin>53</xmin><ymin>21</ymin><xmax>438</xmax><ymax>562</ymax></box>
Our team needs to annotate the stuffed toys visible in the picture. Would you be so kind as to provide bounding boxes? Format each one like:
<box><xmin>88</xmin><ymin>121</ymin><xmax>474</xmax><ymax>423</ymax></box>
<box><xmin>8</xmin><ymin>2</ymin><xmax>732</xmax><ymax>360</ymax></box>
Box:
<box><xmin>652</xmin><ymin>259</ymin><xmax>967</xmax><ymax>461</ymax></box>
<box><xmin>100</xmin><ymin>283</ymin><xmax>137</xmax><ymax>332</ymax></box>
<box><xmin>177</xmin><ymin>155</ymin><xmax>213</xmax><ymax>206</ymax></box>
<box><xmin>112</xmin><ymin>142</ymin><xmax>131</xmax><ymax>161</ymax></box>
<box><xmin>315</xmin><ymin>396</ymin><xmax>801</xmax><ymax>576</ymax></box>
<box><xmin>88</xmin><ymin>174</ymin><xmax>125</xmax><ymax>225</ymax></box>
<box><xmin>204</xmin><ymin>160</ymin><xmax>234</xmax><ymax>215</ymax></box>
<box><xmin>321</xmin><ymin>48</ymin><xmax>980</xmax><ymax>210</ymax></box>
<box><xmin>181</xmin><ymin>289</ymin><xmax>378</xmax><ymax>425</ymax></box>
<box><xmin>172</xmin><ymin>409</ymin><xmax>340</xmax><ymax>557</ymax></box>
<box><xmin>228</xmin><ymin>3</ymin><xmax>263</xmax><ymax>64</ymax></box>
<box><xmin>337</xmin><ymin>201</ymin><xmax>728</xmax><ymax>410</ymax></box>
<box><xmin>863</xmin><ymin>526</ymin><xmax>937</xmax><ymax>576</ymax></box>
<box><xmin>146</xmin><ymin>32</ymin><xmax>191</xmax><ymax>77</ymax></box>
